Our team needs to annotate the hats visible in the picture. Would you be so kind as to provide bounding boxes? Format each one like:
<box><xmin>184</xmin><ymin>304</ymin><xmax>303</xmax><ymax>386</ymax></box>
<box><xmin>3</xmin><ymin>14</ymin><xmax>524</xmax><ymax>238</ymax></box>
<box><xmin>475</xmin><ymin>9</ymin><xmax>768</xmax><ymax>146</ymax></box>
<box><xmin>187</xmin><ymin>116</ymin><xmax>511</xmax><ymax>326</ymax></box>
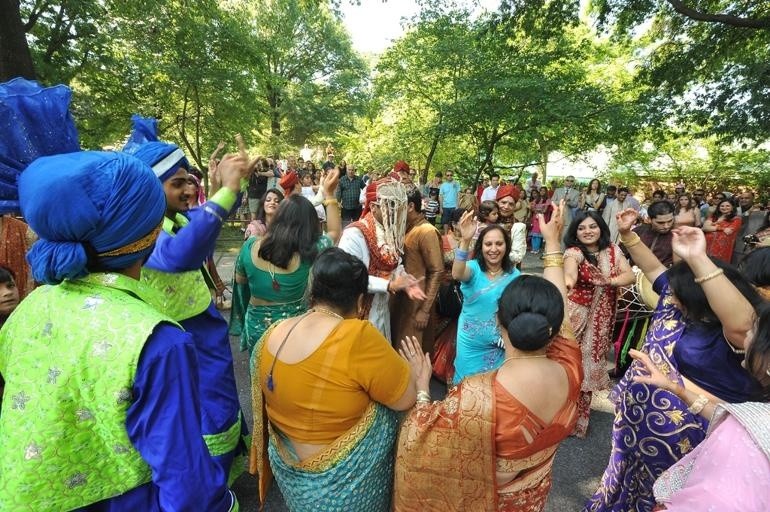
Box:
<box><xmin>495</xmin><ymin>184</ymin><xmax>521</xmax><ymax>203</ymax></box>
<box><xmin>279</xmin><ymin>170</ymin><xmax>298</xmax><ymax>189</ymax></box>
<box><xmin>394</xmin><ymin>160</ymin><xmax>410</xmax><ymax>176</ymax></box>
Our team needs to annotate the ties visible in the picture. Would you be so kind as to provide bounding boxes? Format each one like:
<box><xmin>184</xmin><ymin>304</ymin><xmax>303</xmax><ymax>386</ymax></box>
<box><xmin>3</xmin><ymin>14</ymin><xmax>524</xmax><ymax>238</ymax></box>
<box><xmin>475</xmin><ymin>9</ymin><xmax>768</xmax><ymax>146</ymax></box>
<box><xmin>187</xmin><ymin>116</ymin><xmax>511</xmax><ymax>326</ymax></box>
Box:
<box><xmin>649</xmin><ymin>231</ymin><xmax>661</xmax><ymax>254</ymax></box>
<box><xmin>564</xmin><ymin>188</ymin><xmax>569</xmax><ymax>198</ymax></box>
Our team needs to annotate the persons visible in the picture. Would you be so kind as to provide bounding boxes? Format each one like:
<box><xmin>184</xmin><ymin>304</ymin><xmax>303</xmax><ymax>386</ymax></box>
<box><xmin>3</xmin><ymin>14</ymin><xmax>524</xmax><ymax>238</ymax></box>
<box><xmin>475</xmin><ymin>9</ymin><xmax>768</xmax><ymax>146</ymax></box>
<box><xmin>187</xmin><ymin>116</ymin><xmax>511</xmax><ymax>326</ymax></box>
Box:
<box><xmin>0</xmin><ymin>147</ymin><xmax>241</xmax><ymax>510</ymax></box>
<box><xmin>625</xmin><ymin>299</ymin><xmax>770</xmax><ymax>510</ymax></box>
<box><xmin>2</xmin><ymin>132</ymin><xmax>770</xmax><ymax>440</ymax></box>
<box><xmin>243</xmin><ymin>248</ymin><xmax>419</xmax><ymax>511</ymax></box>
<box><xmin>121</xmin><ymin>112</ymin><xmax>254</xmax><ymax>488</ymax></box>
<box><xmin>585</xmin><ymin>206</ymin><xmax>757</xmax><ymax>511</ymax></box>
<box><xmin>389</xmin><ymin>197</ymin><xmax>586</xmax><ymax>512</ymax></box>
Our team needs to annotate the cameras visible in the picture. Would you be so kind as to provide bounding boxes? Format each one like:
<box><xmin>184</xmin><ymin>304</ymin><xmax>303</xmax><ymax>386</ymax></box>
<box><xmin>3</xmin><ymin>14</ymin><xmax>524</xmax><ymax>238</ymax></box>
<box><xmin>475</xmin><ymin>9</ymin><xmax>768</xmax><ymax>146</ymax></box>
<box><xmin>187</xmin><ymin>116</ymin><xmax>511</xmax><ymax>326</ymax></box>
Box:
<box><xmin>749</xmin><ymin>235</ymin><xmax>758</xmax><ymax>244</ymax></box>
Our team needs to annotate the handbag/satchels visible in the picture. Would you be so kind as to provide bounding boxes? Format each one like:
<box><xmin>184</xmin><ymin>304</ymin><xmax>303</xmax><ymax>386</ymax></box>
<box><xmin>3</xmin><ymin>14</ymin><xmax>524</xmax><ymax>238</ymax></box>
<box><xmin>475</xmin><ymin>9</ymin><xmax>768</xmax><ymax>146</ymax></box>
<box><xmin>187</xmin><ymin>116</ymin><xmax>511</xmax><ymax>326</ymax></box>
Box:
<box><xmin>429</xmin><ymin>318</ymin><xmax>462</xmax><ymax>386</ymax></box>
<box><xmin>435</xmin><ymin>263</ymin><xmax>465</xmax><ymax>316</ymax></box>
